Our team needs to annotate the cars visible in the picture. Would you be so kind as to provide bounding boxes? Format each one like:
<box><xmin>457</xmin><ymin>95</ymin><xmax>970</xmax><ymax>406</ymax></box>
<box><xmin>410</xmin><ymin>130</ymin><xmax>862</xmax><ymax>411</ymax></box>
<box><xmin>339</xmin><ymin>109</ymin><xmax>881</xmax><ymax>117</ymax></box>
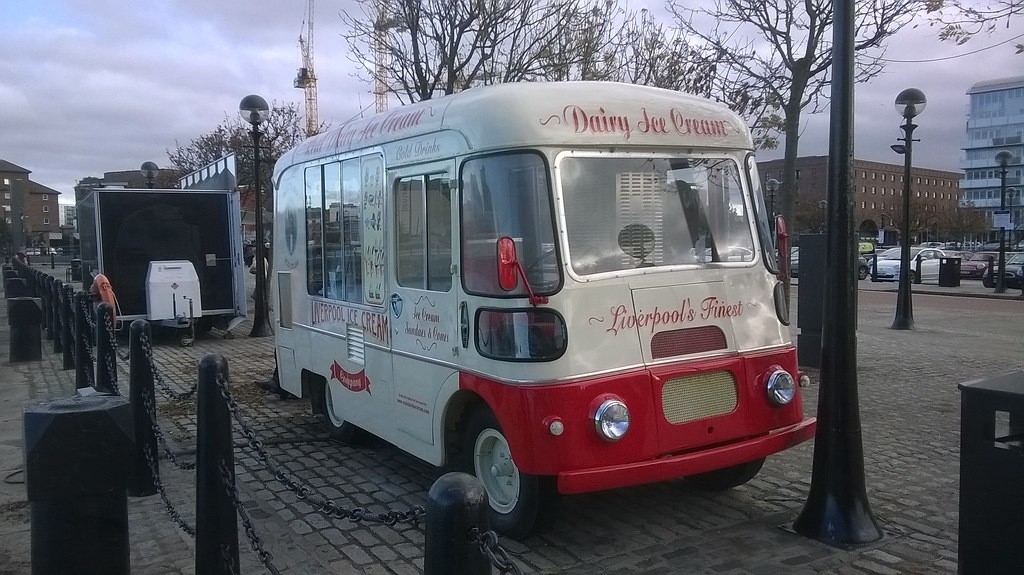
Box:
<box><xmin>774</xmin><ymin>245</ymin><xmax>870</xmax><ymax>280</ymax></box>
<box><xmin>693</xmin><ymin>246</ymin><xmax>754</xmax><ymax>262</ymax></box>
<box><xmin>868</xmin><ymin>240</ymin><xmax>1024</xmax><ymax>290</ymax></box>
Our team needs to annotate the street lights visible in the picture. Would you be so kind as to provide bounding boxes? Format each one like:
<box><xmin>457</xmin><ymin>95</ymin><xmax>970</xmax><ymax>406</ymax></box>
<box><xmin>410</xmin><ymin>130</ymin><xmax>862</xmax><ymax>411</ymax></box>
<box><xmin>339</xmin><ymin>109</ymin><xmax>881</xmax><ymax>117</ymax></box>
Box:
<box><xmin>891</xmin><ymin>88</ymin><xmax>928</xmax><ymax>329</ymax></box>
<box><xmin>1006</xmin><ymin>187</ymin><xmax>1017</xmax><ymax>252</ymax></box>
<box><xmin>240</xmin><ymin>95</ymin><xmax>274</xmax><ymax>337</ymax></box>
<box><xmin>994</xmin><ymin>151</ymin><xmax>1014</xmax><ymax>293</ymax></box>
<box><xmin>766</xmin><ymin>178</ymin><xmax>780</xmax><ymax>230</ymax></box>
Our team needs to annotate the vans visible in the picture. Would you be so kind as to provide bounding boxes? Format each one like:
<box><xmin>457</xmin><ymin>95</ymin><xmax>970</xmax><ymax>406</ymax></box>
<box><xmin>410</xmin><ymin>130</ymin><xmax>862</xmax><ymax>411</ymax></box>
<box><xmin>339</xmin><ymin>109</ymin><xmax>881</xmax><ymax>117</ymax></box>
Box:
<box><xmin>271</xmin><ymin>80</ymin><xmax>819</xmax><ymax>541</ymax></box>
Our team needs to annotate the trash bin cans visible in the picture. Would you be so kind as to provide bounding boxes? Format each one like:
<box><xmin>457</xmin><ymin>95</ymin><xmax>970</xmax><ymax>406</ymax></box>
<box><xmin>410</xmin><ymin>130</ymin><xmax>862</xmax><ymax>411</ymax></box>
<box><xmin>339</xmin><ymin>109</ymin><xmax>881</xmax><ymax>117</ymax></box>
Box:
<box><xmin>71</xmin><ymin>258</ymin><xmax>82</xmax><ymax>280</ymax></box>
<box><xmin>938</xmin><ymin>257</ymin><xmax>961</xmax><ymax>286</ymax></box>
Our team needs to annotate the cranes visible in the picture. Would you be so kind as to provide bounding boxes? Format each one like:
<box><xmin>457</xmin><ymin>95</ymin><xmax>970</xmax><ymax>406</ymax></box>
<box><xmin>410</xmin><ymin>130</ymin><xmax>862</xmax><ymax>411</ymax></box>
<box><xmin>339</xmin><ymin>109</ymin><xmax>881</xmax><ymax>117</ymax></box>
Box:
<box><xmin>374</xmin><ymin>0</ymin><xmax>388</xmax><ymax>114</ymax></box>
<box><xmin>292</xmin><ymin>0</ymin><xmax>317</xmax><ymax>137</ymax></box>
